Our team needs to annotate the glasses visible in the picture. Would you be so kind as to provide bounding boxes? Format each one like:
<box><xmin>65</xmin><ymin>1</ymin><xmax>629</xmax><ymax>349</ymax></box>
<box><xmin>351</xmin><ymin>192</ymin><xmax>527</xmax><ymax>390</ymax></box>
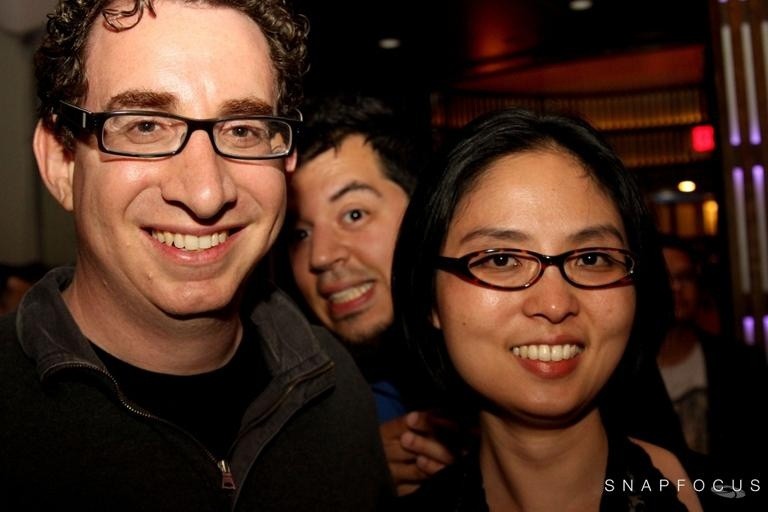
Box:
<box><xmin>51</xmin><ymin>99</ymin><xmax>307</xmax><ymax>160</ymax></box>
<box><xmin>432</xmin><ymin>246</ymin><xmax>636</xmax><ymax>287</ymax></box>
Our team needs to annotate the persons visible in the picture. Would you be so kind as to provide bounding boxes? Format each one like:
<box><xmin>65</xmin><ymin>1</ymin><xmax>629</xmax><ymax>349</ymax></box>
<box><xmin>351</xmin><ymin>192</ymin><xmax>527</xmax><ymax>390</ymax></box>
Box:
<box><xmin>0</xmin><ymin>0</ymin><xmax>400</xmax><ymax>512</ymax></box>
<box><xmin>389</xmin><ymin>105</ymin><xmax>704</xmax><ymax>512</ymax></box>
<box><xmin>278</xmin><ymin>102</ymin><xmax>456</xmax><ymax>497</ymax></box>
<box><xmin>654</xmin><ymin>236</ymin><xmax>723</xmax><ymax>455</ymax></box>
